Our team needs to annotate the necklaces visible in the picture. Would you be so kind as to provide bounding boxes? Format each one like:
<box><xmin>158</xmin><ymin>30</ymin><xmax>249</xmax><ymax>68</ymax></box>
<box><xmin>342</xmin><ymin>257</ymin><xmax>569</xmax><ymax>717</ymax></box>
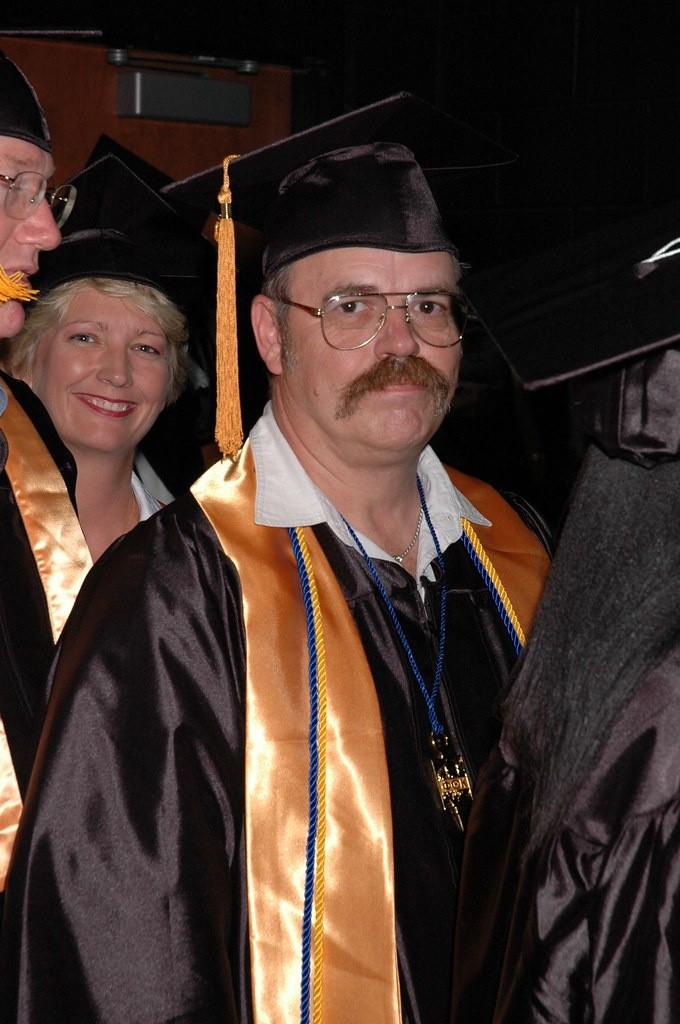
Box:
<box><xmin>392</xmin><ymin>504</ymin><xmax>423</xmax><ymax>562</ymax></box>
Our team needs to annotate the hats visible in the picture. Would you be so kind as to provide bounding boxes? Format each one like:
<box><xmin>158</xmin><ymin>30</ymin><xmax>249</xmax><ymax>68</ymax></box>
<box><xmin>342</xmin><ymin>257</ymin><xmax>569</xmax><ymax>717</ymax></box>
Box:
<box><xmin>22</xmin><ymin>152</ymin><xmax>190</xmax><ymax>316</ymax></box>
<box><xmin>0</xmin><ymin>50</ymin><xmax>53</xmax><ymax>154</ymax></box>
<box><xmin>453</xmin><ymin>197</ymin><xmax>680</xmax><ymax>391</ymax></box>
<box><xmin>158</xmin><ymin>90</ymin><xmax>520</xmax><ymax>459</ymax></box>
<box><xmin>85</xmin><ymin>133</ymin><xmax>176</xmax><ymax>192</ymax></box>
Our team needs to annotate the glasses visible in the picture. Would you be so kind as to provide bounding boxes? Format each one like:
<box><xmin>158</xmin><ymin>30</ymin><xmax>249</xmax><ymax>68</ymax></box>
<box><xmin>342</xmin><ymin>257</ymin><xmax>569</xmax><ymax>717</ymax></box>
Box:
<box><xmin>0</xmin><ymin>170</ymin><xmax>78</xmax><ymax>229</ymax></box>
<box><xmin>262</xmin><ymin>291</ymin><xmax>469</xmax><ymax>352</ymax></box>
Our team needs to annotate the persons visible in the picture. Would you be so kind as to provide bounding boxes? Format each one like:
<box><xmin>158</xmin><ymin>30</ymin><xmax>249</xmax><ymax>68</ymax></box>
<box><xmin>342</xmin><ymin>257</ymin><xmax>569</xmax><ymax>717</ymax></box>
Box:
<box><xmin>0</xmin><ymin>31</ymin><xmax>258</xmax><ymax>1023</ymax></box>
<box><xmin>0</xmin><ymin>88</ymin><xmax>570</xmax><ymax>1024</ymax></box>
<box><xmin>450</xmin><ymin>208</ymin><xmax>680</xmax><ymax>1023</ymax></box>
